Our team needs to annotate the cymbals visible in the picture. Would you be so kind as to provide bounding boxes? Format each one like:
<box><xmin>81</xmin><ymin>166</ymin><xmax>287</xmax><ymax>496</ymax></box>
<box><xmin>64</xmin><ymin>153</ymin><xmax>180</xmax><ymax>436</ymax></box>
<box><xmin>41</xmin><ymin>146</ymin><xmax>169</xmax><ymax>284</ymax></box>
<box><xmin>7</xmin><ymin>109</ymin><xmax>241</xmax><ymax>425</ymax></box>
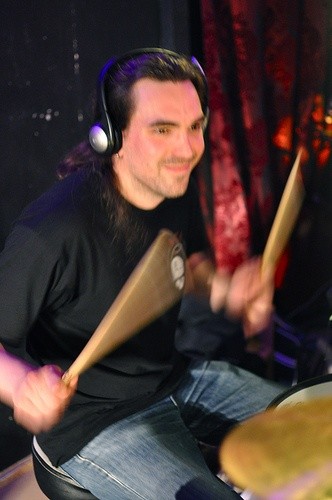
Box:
<box><xmin>218</xmin><ymin>397</ymin><xmax>332</xmax><ymax>499</ymax></box>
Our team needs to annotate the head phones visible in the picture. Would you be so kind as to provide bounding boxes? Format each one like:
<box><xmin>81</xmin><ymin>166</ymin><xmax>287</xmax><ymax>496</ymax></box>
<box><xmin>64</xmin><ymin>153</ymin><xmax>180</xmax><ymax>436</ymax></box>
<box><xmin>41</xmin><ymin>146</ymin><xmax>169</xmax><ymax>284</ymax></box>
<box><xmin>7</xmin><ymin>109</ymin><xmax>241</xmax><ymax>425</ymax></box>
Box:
<box><xmin>88</xmin><ymin>48</ymin><xmax>210</xmax><ymax>157</ymax></box>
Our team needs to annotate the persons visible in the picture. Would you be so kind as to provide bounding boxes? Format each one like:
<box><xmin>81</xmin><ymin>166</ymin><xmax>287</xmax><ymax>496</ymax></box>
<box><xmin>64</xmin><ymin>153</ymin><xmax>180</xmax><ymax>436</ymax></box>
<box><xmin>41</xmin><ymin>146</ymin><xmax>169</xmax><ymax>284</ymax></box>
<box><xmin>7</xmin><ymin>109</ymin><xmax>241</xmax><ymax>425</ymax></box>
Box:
<box><xmin>0</xmin><ymin>47</ymin><xmax>307</xmax><ymax>500</ymax></box>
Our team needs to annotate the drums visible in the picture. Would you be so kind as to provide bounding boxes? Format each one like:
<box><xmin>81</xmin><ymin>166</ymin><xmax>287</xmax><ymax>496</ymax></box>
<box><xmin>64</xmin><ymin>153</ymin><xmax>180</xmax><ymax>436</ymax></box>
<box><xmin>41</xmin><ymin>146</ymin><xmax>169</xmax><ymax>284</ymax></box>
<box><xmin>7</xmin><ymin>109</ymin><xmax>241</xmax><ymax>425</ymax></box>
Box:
<box><xmin>265</xmin><ymin>375</ymin><xmax>332</xmax><ymax>416</ymax></box>
